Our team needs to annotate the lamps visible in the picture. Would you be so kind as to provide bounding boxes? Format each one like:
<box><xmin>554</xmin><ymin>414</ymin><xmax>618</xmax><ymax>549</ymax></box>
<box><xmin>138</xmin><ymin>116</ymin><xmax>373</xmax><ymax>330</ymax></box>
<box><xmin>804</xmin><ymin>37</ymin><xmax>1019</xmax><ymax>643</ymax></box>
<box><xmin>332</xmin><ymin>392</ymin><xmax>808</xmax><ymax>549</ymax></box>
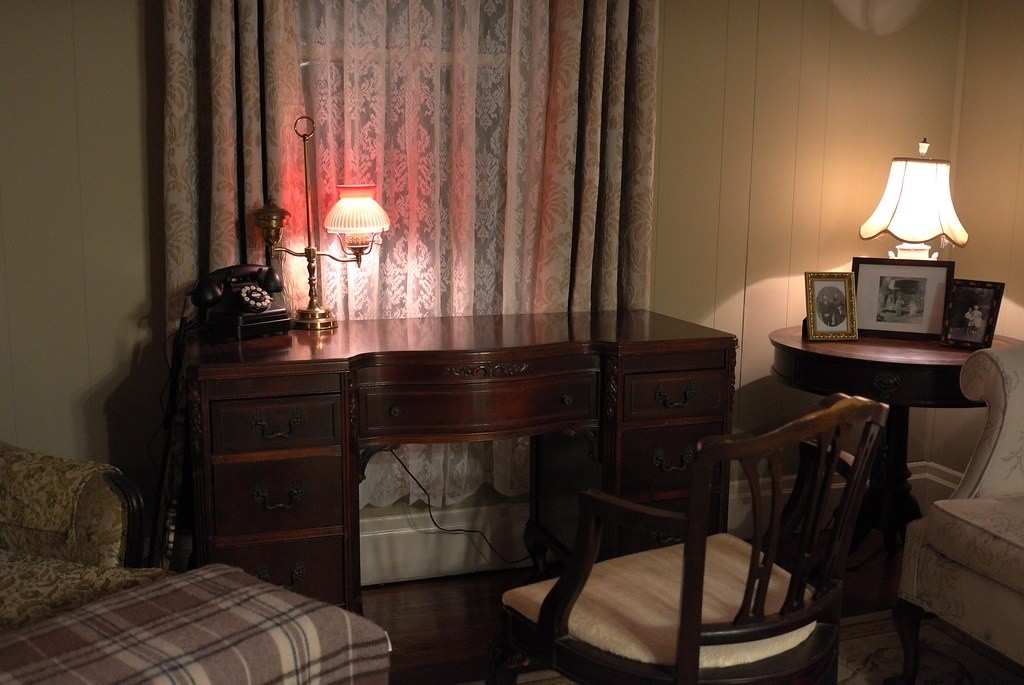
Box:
<box><xmin>251</xmin><ymin>115</ymin><xmax>393</xmax><ymax>329</ymax></box>
<box><xmin>857</xmin><ymin>137</ymin><xmax>971</xmax><ymax>260</ymax></box>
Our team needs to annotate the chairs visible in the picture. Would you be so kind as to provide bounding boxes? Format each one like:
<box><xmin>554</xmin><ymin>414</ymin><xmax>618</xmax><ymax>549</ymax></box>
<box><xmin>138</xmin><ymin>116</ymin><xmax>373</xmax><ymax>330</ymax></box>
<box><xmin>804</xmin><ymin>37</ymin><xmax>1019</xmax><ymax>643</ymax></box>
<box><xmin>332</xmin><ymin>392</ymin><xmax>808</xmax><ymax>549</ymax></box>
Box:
<box><xmin>891</xmin><ymin>344</ymin><xmax>1024</xmax><ymax>685</ymax></box>
<box><xmin>489</xmin><ymin>393</ymin><xmax>890</xmax><ymax>685</ymax></box>
<box><xmin>0</xmin><ymin>440</ymin><xmax>178</xmax><ymax>640</ymax></box>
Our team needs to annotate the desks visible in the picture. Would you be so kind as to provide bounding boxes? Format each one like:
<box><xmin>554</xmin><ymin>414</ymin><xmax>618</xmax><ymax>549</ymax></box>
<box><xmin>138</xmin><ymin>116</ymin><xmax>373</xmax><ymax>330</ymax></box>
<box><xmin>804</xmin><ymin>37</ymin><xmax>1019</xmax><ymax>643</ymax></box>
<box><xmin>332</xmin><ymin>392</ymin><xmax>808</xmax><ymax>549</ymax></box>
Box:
<box><xmin>770</xmin><ymin>323</ymin><xmax>1024</xmax><ymax>609</ymax></box>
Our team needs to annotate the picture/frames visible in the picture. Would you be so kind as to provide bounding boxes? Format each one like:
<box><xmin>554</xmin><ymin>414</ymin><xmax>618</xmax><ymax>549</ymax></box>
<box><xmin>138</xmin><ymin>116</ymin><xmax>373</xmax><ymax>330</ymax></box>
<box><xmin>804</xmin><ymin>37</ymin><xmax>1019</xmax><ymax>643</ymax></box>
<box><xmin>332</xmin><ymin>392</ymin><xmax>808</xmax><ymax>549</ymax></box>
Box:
<box><xmin>852</xmin><ymin>256</ymin><xmax>955</xmax><ymax>341</ymax></box>
<box><xmin>948</xmin><ymin>278</ymin><xmax>1005</xmax><ymax>351</ymax></box>
<box><xmin>803</xmin><ymin>271</ymin><xmax>859</xmax><ymax>342</ymax></box>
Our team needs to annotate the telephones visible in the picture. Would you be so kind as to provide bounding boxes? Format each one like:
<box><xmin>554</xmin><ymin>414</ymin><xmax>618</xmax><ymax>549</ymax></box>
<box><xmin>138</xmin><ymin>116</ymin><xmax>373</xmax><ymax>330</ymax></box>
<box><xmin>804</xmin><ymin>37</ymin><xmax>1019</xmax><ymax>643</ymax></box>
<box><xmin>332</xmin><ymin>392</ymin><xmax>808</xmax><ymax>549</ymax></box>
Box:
<box><xmin>189</xmin><ymin>264</ymin><xmax>292</xmax><ymax>340</ymax></box>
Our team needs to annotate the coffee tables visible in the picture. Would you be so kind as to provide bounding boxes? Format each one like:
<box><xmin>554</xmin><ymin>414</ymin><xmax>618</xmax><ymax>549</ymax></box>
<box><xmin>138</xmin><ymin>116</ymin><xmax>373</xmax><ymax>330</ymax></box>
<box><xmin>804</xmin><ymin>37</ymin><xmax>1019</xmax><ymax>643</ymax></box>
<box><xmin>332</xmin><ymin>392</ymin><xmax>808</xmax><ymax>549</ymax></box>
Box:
<box><xmin>0</xmin><ymin>563</ymin><xmax>393</xmax><ymax>685</ymax></box>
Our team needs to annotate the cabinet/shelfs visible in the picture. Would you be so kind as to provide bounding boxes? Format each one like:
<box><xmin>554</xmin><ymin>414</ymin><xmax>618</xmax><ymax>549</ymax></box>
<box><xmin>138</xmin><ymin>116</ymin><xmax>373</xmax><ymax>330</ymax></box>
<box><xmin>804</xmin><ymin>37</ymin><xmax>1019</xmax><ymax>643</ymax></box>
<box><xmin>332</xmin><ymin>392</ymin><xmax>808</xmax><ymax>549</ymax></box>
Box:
<box><xmin>190</xmin><ymin>308</ymin><xmax>739</xmax><ymax>617</ymax></box>
